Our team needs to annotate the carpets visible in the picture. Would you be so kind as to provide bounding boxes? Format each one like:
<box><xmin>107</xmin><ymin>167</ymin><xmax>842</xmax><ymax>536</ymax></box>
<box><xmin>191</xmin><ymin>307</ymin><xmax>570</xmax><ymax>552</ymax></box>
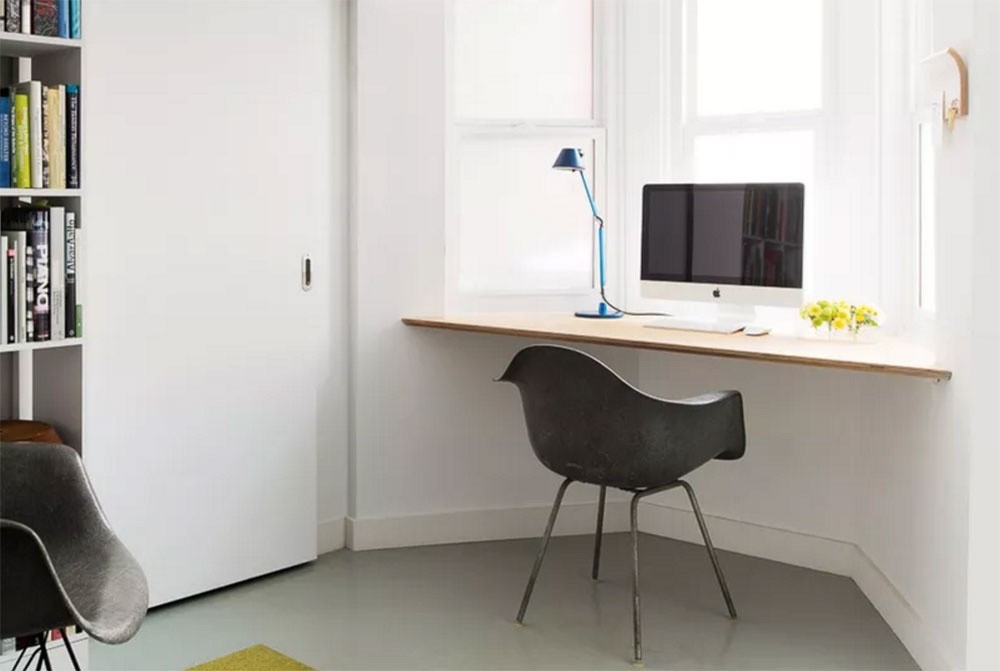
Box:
<box><xmin>184</xmin><ymin>644</ymin><xmax>319</xmax><ymax>671</ymax></box>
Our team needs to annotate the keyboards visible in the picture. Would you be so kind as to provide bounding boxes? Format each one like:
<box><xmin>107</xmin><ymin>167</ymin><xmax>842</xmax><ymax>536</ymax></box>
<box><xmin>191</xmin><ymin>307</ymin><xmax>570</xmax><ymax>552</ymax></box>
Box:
<box><xmin>643</xmin><ymin>315</ymin><xmax>748</xmax><ymax>334</ymax></box>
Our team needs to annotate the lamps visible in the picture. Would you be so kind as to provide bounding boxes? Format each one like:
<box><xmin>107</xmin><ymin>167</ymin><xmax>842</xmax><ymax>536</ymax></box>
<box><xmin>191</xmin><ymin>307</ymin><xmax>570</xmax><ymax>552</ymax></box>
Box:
<box><xmin>552</xmin><ymin>146</ymin><xmax>625</xmax><ymax>320</ymax></box>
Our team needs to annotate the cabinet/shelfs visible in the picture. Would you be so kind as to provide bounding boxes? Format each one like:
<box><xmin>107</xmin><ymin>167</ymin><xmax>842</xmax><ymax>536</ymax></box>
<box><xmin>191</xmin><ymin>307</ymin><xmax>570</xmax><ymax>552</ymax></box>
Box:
<box><xmin>0</xmin><ymin>442</ymin><xmax>153</xmax><ymax>671</ymax></box>
<box><xmin>0</xmin><ymin>0</ymin><xmax>88</xmax><ymax>662</ymax></box>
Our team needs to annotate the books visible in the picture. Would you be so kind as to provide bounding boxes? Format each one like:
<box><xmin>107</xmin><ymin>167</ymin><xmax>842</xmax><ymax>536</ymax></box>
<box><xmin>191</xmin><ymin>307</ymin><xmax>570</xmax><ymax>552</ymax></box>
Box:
<box><xmin>0</xmin><ymin>205</ymin><xmax>82</xmax><ymax>344</ymax></box>
<box><xmin>0</xmin><ymin>624</ymin><xmax>83</xmax><ymax>655</ymax></box>
<box><xmin>0</xmin><ymin>55</ymin><xmax>79</xmax><ymax>189</ymax></box>
<box><xmin>0</xmin><ymin>0</ymin><xmax>80</xmax><ymax>39</ymax></box>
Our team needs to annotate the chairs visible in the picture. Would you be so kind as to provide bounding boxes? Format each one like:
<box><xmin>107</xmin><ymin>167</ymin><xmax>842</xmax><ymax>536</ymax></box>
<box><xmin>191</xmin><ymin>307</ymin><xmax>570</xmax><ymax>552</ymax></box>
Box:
<box><xmin>490</xmin><ymin>342</ymin><xmax>746</xmax><ymax>665</ymax></box>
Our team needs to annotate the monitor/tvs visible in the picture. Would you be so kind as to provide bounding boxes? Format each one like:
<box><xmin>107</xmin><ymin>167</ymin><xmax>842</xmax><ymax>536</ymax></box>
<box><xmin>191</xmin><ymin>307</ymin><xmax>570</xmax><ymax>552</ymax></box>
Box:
<box><xmin>641</xmin><ymin>181</ymin><xmax>805</xmax><ymax>319</ymax></box>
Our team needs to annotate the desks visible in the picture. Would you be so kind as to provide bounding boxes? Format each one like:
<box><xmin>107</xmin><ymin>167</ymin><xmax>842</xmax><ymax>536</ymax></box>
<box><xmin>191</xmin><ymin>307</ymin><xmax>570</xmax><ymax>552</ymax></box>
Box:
<box><xmin>400</xmin><ymin>307</ymin><xmax>953</xmax><ymax>381</ymax></box>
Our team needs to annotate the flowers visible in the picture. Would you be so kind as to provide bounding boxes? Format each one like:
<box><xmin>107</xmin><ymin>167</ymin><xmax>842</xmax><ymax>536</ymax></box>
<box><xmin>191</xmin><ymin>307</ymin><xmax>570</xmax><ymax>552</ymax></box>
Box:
<box><xmin>801</xmin><ymin>297</ymin><xmax>879</xmax><ymax>337</ymax></box>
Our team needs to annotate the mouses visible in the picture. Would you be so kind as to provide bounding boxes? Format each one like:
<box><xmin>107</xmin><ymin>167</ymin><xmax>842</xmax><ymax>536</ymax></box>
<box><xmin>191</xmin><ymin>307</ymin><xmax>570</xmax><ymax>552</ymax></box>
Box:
<box><xmin>744</xmin><ymin>324</ymin><xmax>772</xmax><ymax>335</ymax></box>
